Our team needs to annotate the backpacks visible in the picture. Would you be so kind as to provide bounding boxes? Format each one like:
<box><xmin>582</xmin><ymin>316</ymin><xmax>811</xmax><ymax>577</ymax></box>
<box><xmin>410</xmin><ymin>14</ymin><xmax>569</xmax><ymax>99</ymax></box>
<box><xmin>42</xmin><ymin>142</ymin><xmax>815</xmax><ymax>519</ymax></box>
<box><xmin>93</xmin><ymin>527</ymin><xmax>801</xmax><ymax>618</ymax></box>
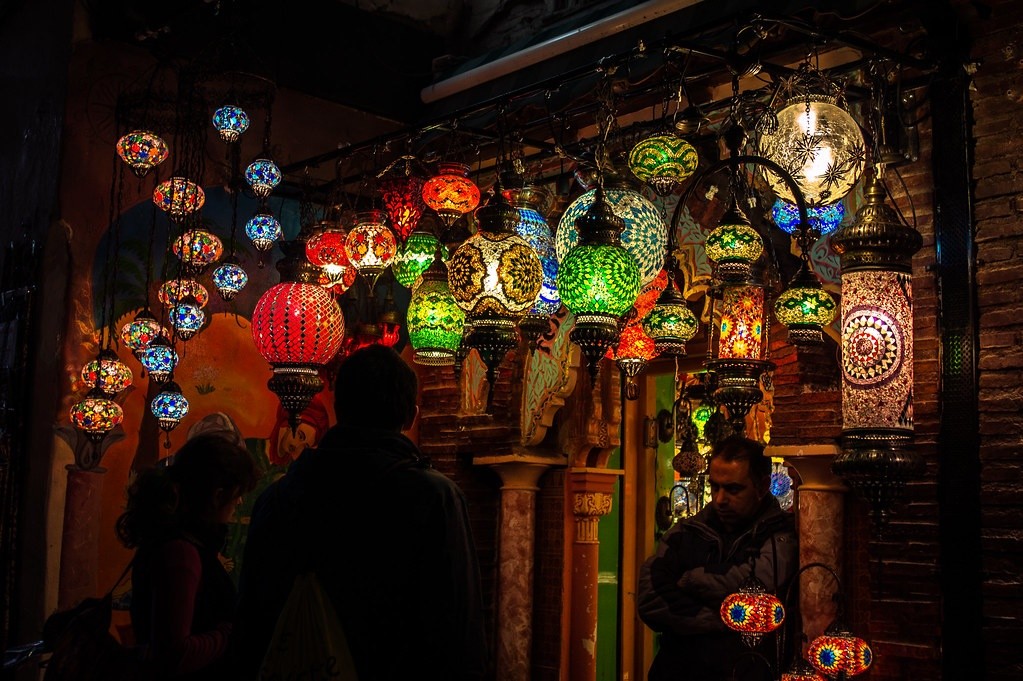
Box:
<box><xmin>257</xmin><ymin>455</ymin><xmax>432</xmax><ymax>681</ymax></box>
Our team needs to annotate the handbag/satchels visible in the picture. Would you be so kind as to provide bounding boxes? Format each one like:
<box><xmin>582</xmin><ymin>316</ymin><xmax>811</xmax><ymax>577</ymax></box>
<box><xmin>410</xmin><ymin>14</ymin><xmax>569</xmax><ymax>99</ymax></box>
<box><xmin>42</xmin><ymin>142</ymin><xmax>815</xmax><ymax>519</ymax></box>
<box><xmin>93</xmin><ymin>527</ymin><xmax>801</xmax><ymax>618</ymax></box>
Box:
<box><xmin>43</xmin><ymin>530</ymin><xmax>203</xmax><ymax>681</ymax></box>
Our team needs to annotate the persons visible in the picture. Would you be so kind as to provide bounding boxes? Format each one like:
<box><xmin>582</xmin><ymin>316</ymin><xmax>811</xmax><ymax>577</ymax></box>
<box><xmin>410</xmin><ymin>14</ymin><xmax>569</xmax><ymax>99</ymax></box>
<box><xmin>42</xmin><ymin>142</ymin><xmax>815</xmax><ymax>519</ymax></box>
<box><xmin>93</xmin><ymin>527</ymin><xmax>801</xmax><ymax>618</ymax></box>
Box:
<box><xmin>116</xmin><ymin>428</ymin><xmax>265</xmax><ymax>681</ymax></box>
<box><xmin>636</xmin><ymin>435</ymin><xmax>798</xmax><ymax>681</ymax></box>
<box><xmin>236</xmin><ymin>343</ymin><xmax>491</xmax><ymax>681</ymax></box>
